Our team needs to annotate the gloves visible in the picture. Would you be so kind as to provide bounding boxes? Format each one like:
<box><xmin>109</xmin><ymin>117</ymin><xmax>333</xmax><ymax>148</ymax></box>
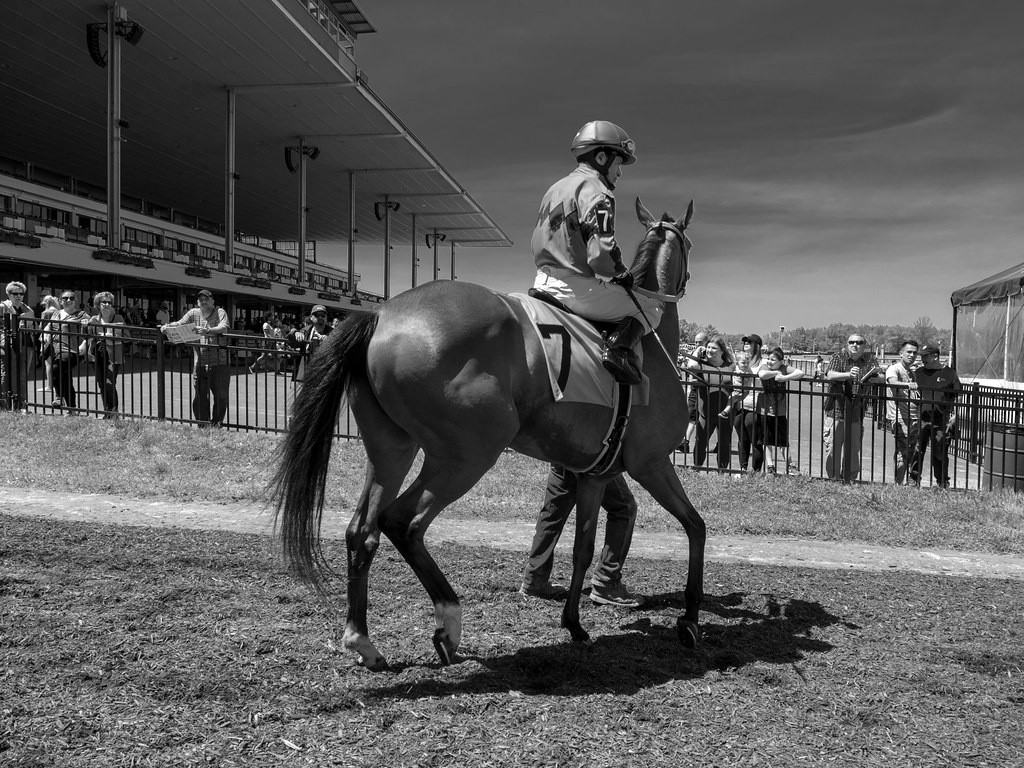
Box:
<box><xmin>612</xmin><ymin>272</ymin><xmax>634</xmax><ymax>290</ymax></box>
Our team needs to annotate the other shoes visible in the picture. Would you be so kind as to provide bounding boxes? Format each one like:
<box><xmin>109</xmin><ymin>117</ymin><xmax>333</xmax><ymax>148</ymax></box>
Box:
<box><xmin>249</xmin><ymin>366</ymin><xmax>255</xmax><ymax>373</ymax></box>
<box><xmin>718</xmin><ymin>410</ymin><xmax>728</xmax><ymax>419</ymax></box>
<box><xmin>766</xmin><ymin>465</ymin><xmax>775</xmax><ymax>473</ymax></box>
<box><xmin>735</xmin><ymin>409</ymin><xmax>744</xmax><ymax>417</ymax></box>
<box><xmin>816</xmin><ymin>383</ymin><xmax>822</xmax><ymax>387</ymax></box>
<box><xmin>52</xmin><ymin>397</ymin><xmax>78</xmax><ymax>418</ymax></box>
<box><xmin>710</xmin><ymin>442</ymin><xmax>719</xmax><ymax>453</ymax></box>
<box><xmin>676</xmin><ymin>439</ymin><xmax>690</xmax><ymax>453</ymax></box>
<box><xmin>277</xmin><ymin>370</ymin><xmax>283</xmax><ymax>375</ymax></box>
<box><xmin>789</xmin><ymin>463</ymin><xmax>800</xmax><ymax>473</ymax></box>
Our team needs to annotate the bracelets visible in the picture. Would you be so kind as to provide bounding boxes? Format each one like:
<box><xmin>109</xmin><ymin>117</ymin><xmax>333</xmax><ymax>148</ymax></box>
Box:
<box><xmin>207</xmin><ymin>328</ymin><xmax>210</xmax><ymax>334</ymax></box>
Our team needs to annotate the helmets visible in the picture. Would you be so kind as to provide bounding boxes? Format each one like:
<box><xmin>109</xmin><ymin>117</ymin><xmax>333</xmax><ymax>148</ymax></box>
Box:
<box><xmin>569</xmin><ymin>121</ymin><xmax>637</xmax><ymax>166</ymax></box>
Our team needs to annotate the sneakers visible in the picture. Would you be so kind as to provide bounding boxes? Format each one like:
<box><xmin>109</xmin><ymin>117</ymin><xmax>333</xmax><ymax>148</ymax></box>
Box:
<box><xmin>590</xmin><ymin>580</ymin><xmax>645</xmax><ymax>607</ymax></box>
<box><xmin>518</xmin><ymin>582</ymin><xmax>567</xmax><ymax>601</ymax></box>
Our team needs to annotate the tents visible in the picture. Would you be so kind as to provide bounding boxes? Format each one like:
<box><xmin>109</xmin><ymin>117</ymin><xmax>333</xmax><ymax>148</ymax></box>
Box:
<box><xmin>949</xmin><ymin>262</ymin><xmax>1024</xmax><ymax>380</ymax></box>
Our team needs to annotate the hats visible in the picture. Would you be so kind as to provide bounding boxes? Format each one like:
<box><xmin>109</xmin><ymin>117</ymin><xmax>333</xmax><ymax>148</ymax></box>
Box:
<box><xmin>742</xmin><ymin>334</ymin><xmax>762</xmax><ymax>346</ymax></box>
<box><xmin>197</xmin><ymin>290</ymin><xmax>213</xmax><ymax>298</ymax></box>
<box><xmin>917</xmin><ymin>345</ymin><xmax>940</xmax><ymax>355</ymax></box>
<box><xmin>311</xmin><ymin>305</ymin><xmax>328</xmax><ymax>315</ymax></box>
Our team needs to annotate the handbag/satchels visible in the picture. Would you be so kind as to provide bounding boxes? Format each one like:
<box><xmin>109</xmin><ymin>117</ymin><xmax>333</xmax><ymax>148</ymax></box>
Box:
<box><xmin>39</xmin><ymin>342</ymin><xmax>52</xmax><ymax>361</ymax></box>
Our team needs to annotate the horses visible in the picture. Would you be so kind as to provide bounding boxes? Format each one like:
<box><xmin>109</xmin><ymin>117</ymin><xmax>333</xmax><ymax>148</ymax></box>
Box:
<box><xmin>250</xmin><ymin>197</ymin><xmax>707</xmax><ymax>675</ymax></box>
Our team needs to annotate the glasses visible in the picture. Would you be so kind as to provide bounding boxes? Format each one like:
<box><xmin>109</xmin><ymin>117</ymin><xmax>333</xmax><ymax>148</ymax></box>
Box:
<box><xmin>10</xmin><ymin>292</ymin><xmax>24</xmax><ymax>296</ymax></box>
<box><xmin>100</xmin><ymin>301</ymin><xmax>112</xmax><ymax>305</ymax></box>
<box><xmin>847</xmin><ymin>341</ymin><xmax>864</xmax><ymax>345</ymax></box>
<box><xmin>62</xmin><ymin>297</ymin><xmax>75</xmax><ymax>301</ymax></box>
<box><xmin>314</xmin><ymin>313</ymin><xmax>325</xmax><ymax>318</ymax></box>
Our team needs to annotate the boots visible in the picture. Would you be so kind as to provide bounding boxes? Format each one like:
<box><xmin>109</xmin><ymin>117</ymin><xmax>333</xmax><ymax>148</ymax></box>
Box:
<box><xmin>602</xmin><ymin>316</ymin><xmax>645</xmax><ymax>384</ymax></box>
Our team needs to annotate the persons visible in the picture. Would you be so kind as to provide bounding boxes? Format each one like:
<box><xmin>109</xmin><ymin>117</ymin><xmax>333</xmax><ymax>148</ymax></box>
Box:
<box><xmin>519</xmin><ymin>462</ymin><xmax>645</xmax><ymax>608</ymax></box>
<box><xmin>0</xmin><ymin>281</ymin><xmax>39</xmax><ymax>415</ymax></box>
<box><xmin>236</xmin><ymin>311</ymin><xmax>339</xmax><ymax>376</ymax></box>
<box><xmin>884</xmin><ymin>340</ymin><xmax>963</xmax><ymax>488</ymax></box>
<box><xmin>160</xmin><ymin>290</ymin><xmax>231</xmax><ymax>429</ymax></box>
<box><xmin>823</xmin><ymin>333</ymin><xmax>885</xmax><ymax>478</ymax></box>
<box><xmin>674</xmin><ymin>332</ymin><xmax>823</xmax><ymax>479</ymax></box>
<box><xmin>38</xmin><ymin>290</ymin><xmax>170</xmax><ymax>420</ymax></box>
<box><xmin>286</xmin><ymin>304</ymin><xmax>339</xmax><ymax>445</ymax></box>
<box><xmin>531</xmin><ymin>120</ymin><xmax>663</xmax><ymax>385</ymax></box>
